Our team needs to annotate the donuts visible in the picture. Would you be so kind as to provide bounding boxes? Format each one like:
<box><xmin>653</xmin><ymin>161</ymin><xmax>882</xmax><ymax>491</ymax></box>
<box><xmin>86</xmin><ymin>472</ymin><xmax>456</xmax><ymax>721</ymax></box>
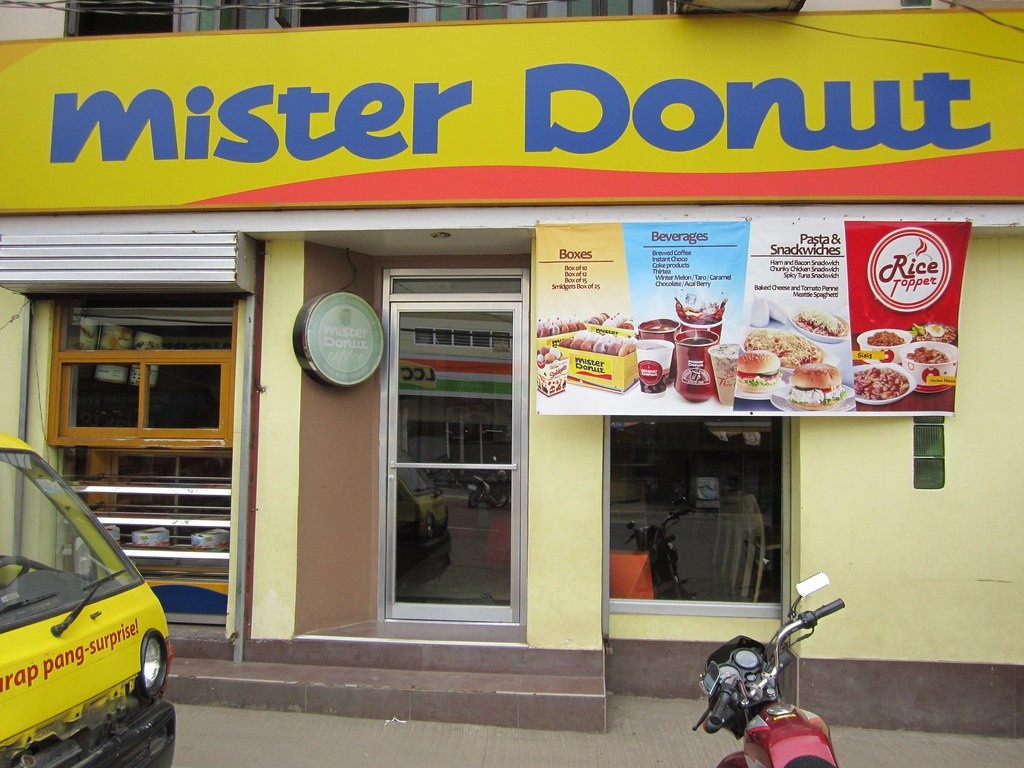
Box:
<box><xmin>537</xmin><ymin>313</ymin><xmax>636</xmax><ymax>357</ymax></box>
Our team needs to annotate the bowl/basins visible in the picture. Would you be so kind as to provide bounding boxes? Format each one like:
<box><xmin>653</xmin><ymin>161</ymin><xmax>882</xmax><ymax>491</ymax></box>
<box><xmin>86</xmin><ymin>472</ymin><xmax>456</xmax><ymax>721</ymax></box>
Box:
<box><xmin>853</xmin><ymin>364</ymin><xmax>917</xmax><ymax>405</ymax></box>
<box><xmin>901</xmin><ymin>342</ymin><xmax>959</xmax><ymax>393</ymax></box>
<box><xmin>857</xmin><ymin>329</ymin><xmax>912</xmax><ymax>367</ymax></box>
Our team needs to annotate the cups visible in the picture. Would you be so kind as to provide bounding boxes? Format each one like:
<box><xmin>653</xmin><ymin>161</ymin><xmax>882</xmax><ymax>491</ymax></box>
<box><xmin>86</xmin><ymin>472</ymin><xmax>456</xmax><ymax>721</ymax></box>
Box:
<box><xmin>638</xmin><ymin>319</ymin><xmax>681</xmax><ymax>376</ymax></box>
<box><xmin>675</xmin><ymin>330</ymin><xmax>720</xmax><ymax>402</ymax></box>
<box><xmin>708</xmin><ymin>344</ymin><xmax>740</xmax><ymax>406</ymax></box>
<box><xmin>750</xmin><ymin>295</ymin><xmax>769</xmax><ymax>327</ymax></box>
<box><xmin>636</xmin><ymin>339</ymin><xmax>676</xmax><ymax>393</ymax></box>
<box><xmin>678</xmin><ymin>317</ymin><xmax>723</xmax><ymax>338</ymax></box>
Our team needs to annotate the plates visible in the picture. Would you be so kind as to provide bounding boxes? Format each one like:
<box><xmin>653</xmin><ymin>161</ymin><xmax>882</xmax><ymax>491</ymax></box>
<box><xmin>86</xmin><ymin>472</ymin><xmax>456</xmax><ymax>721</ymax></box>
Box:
<box><xmin>789</xmin><ymin>308</ymin><xmax>849</xmax><ymax>343</ymax></box>
<box><xmin>740</xmin><ymin>336</ymin><xmax>824</xmax><ymax>369</ymax></box>
<box><xmin>909</xmin><ymin>322</ymin><xmax>958</xmax><ymax>346</ymax></box>
<box><xmin>734</xmin><ymin>380</ymin><xmax>785</xmax><ymax>400</ymax></box>
<box><xmin>771</xmin><ymin>384</ymin><xmax>856</xmax><ymax>411</ymax></box>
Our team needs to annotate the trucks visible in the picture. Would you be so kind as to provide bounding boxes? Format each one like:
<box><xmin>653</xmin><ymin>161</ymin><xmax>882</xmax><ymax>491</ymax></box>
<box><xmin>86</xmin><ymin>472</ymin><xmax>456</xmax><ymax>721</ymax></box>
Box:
<box><xmin>395</xmin><ymin>445</ymin><xmax>452</xmax><ymax>556</ymax></box>
<box><xmin>0</xmin><ymin>431</ymin><xmax>178</xmax><ymax>768</ymax></box>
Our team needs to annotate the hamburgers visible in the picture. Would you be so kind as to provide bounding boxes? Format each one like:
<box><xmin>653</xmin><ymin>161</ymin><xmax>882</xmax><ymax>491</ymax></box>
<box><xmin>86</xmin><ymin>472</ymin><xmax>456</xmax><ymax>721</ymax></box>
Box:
<box><xmin>735</xmin><ymin>350</ymin><xmax>784</xmax><ymax>393</ymax></box>
<box><xmin>789</xmin><ymin>362</ymin><xmax>843</xmax><ymax>408</ymax></box>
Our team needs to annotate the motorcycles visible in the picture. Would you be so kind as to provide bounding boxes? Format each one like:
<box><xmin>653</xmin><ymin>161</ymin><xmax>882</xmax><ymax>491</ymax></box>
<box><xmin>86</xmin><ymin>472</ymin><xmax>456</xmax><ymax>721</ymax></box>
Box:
<box><xmin>691</xmin><ymin>571</ymin><xmax>847</xmax><ymax>768</ymax></box>
<box><xmin>622</xmin><ymin>496</ymin><xmax>699</xmax><ymax>601</ymax></box>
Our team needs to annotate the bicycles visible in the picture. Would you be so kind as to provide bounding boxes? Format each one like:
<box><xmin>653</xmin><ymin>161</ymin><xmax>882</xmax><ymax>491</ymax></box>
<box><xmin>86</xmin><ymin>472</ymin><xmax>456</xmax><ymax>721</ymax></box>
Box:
<box><xmin>424</xmin><ymin>452</ymin><xmax>460</xmax><ymax>489</ymax></box>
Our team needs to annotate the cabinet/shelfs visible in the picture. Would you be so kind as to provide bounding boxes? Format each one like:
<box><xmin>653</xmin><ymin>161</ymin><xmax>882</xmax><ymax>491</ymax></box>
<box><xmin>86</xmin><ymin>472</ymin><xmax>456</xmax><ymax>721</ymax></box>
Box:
<box><xmin>57</xmin><ymin>446</ymin><xmax>232</xmax><ymax>625</ymax></box>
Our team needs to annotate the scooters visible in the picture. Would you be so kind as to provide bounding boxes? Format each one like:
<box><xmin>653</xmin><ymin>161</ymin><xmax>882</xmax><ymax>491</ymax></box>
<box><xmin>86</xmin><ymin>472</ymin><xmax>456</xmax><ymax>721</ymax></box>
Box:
<box><xmin>464</xmin><ymin>454</ymin><xmax>509</xmax><ymax>509</ymax></box>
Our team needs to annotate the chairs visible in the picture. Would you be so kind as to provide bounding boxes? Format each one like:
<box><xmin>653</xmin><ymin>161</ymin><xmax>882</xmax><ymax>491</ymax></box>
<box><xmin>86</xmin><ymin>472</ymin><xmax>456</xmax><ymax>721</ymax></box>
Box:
<box><xmin>713</xmin><ymin>489</ymin><xmax>783</xmax><ymax>602</ymax></box>
